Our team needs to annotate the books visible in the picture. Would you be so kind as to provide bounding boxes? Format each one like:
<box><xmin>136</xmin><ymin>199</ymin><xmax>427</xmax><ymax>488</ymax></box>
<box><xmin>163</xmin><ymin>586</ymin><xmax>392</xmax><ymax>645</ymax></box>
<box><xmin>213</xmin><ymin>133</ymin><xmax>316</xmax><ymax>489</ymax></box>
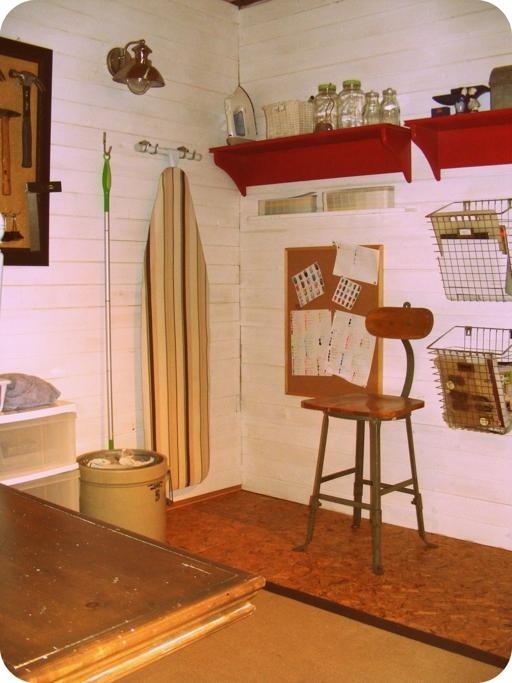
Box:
<box><xmin>495</xmin><ymin>361</ymin><xmax>511</xmax><ymax>425</ymax></box>
<box><xmin>435</xmin><ymin>353</ymin><xmax>509</xmax><ymax>432</ymax></box>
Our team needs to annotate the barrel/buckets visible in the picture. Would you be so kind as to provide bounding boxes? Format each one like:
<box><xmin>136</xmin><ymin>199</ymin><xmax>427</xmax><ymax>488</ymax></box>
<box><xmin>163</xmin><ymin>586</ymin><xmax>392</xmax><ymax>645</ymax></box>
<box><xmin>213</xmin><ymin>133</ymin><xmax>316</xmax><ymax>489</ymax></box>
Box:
<box><xmin>78</xmin><ymin>449</ymin><xmax>167</xmax><ymax>550</ymax></box>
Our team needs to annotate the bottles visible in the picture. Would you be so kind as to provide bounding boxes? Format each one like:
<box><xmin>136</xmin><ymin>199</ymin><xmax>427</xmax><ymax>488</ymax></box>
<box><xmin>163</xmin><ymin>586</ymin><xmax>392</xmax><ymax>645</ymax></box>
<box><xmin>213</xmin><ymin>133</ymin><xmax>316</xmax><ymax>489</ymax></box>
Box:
<box><xmin>314</xmin><ymin>77</ymin><xmax>401</xmax><ymax>128</ymax></box>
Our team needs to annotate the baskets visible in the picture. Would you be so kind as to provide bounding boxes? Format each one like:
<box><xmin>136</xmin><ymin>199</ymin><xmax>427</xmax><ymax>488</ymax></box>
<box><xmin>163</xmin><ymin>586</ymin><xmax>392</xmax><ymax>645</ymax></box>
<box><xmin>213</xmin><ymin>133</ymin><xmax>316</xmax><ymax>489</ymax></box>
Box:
<box><xmin>424</xmin><ymin>199</ymin><xmax>511</xmax><ymax>302</ymax></box>
<box><xmin>426</xmin><ymin>325</ymin><xmax>512</xmax><ymax>436</ymax></box>
<box><xmin>262</xmin><ymin>100</ymin><xmax>317</xmax><ymax>140</ymax></box>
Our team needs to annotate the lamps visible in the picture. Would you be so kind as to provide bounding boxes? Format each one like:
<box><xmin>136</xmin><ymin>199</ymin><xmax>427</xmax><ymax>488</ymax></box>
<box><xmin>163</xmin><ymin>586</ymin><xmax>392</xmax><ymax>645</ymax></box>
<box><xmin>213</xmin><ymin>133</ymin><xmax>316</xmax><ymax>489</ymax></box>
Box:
<box><xmin>105</xmin><ymin>38</ymin><xmax>165</xmax><ymax>94</ymax></box>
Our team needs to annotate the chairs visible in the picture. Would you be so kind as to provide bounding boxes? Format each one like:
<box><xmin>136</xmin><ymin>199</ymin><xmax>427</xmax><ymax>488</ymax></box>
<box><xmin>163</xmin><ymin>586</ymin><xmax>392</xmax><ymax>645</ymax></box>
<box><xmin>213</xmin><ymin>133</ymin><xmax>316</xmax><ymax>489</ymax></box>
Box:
<box><xmin>291</xmin><ymin>301</ymin><xmax>439</xmax><ymax>572</ymax></box>
